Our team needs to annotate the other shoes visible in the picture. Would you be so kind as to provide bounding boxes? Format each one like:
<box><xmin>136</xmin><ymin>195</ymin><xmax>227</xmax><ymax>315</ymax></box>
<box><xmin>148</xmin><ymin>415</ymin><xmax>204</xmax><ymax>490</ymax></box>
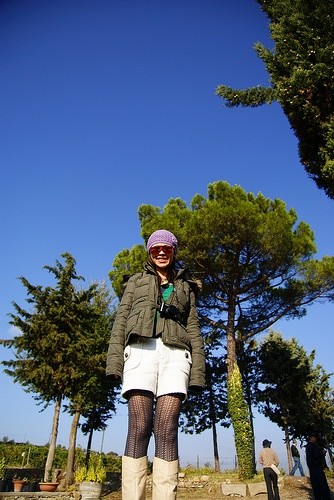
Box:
<box><xmin>290</xmin><ymin>473</ymin><xmax>294</xmax><ymax>476</ymax></box>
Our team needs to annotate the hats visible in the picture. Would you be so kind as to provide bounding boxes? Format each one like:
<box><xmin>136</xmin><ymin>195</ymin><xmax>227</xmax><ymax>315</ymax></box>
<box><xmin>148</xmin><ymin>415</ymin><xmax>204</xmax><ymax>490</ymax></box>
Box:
<box><xmin>262</xmin><ymin>439</ymin><xmax>272</xmax><ymax>448</ymax></box>
<box><xmin>147</xmin><ymin>229</ymin><xmax>177</xmax><ymax>255</ymax></box>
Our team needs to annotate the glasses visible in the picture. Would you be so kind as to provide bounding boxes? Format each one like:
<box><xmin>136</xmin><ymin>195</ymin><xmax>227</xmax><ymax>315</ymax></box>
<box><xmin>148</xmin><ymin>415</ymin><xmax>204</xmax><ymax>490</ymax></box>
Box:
<box><xmin>150</xmin><ymin>245</ymin><xmax>173</xmax><ymax>255</ymax></box>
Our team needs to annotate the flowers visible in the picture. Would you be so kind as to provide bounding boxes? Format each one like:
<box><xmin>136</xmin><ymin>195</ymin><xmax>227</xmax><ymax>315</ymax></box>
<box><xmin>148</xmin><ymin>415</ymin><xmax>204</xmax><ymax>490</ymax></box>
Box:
<box><xmin>28</xmin><ymin>473</ymin><xmax>37</xmax><ymax>485</ymax></box>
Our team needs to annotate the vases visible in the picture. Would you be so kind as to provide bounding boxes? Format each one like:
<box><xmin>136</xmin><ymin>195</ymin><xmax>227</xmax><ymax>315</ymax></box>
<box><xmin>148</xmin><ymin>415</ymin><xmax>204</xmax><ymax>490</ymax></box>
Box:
<box><xmin>28</xmin><ymin>481</ymin><xmax>37</xmax><ymax>492</ymax></box>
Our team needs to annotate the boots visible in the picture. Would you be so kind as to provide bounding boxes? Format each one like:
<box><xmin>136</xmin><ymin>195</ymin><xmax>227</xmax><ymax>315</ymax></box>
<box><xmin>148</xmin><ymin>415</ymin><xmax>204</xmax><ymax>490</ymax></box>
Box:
<box><xmin>122</xmin><ymin>455</ymin><xmax>148</xmax><ymax>500</ymax></box>
<box><xmin>152</xmin><ymin>456</ymin><xmax>179</xmax><ymax>500</ymax></box>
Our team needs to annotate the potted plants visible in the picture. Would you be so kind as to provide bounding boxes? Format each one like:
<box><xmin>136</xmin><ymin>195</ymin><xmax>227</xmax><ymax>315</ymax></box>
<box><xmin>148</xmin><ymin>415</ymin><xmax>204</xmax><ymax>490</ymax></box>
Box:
<box><xmin>74</xmin><ymin>455</ymin><xmax>106</xmax><ymax>500</ymax></box>
<box><xmin>38</xmin><ymin>468</ymin><xmax>59</xmax><ymax>492</ymax></box>
<box><xmin>12</xmin><ymin>475</ymin><xmax>28</xmax><ymax>492</ymax></box>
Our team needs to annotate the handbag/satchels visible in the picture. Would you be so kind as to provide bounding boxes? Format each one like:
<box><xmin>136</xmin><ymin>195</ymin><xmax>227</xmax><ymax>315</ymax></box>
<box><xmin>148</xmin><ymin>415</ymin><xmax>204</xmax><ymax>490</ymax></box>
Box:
<box><xmin>270</xmin><ymin>464</ymin><xmax>280</xmax><ymax>474</ymax></box>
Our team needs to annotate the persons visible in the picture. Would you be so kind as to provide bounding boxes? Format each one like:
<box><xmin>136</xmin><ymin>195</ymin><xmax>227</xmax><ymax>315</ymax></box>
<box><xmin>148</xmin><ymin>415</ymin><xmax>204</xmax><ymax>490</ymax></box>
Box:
<box><xmin>289</xmin><ymin>440</ymin><xmax>305</xmax><ymax>476</ymax></box>
<box><xmin>259</xmin><ymin>440</ymin><xmax>280</xmax><ymax>500</ymax></box>
<box><xmin>306</xmin><ymin>434</ymin><xmax>330</xmax><ymax>500</ymax></box>
<box><xmin>107</xmin><ymin>230</ymin><xmax>206</xmax><ymax>500</ymax></box>
